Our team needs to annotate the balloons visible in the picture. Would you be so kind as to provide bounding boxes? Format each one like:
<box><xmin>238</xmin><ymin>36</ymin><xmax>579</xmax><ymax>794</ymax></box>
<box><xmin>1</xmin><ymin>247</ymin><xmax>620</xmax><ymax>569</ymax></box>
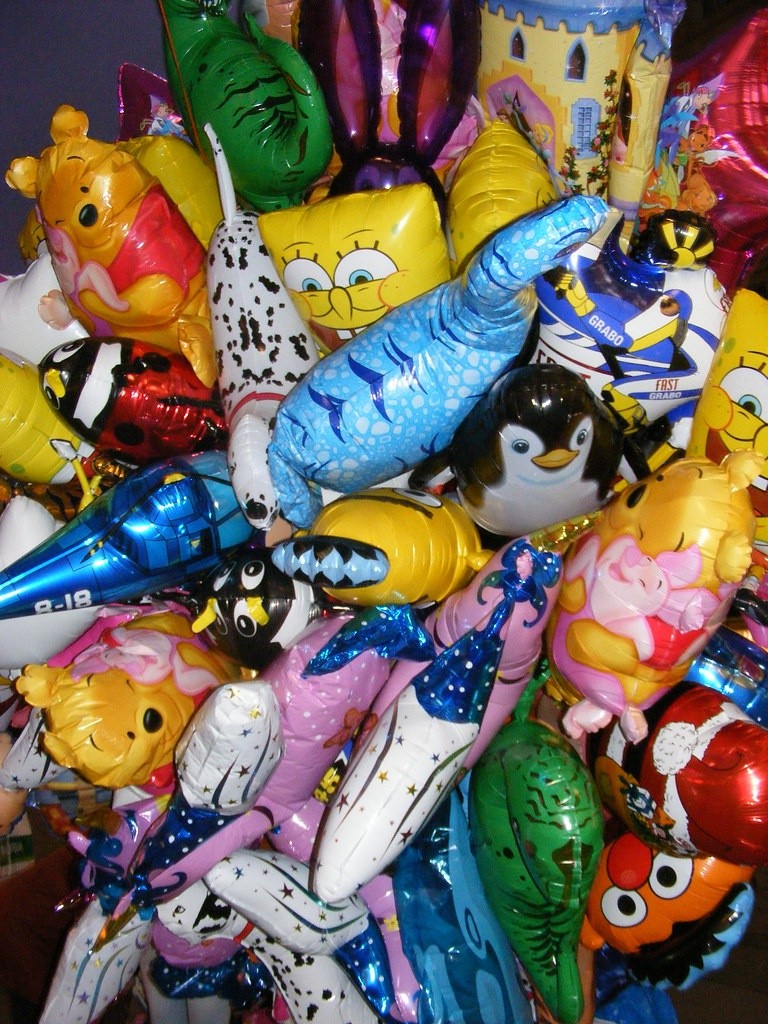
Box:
<box><xmin>0</xmin><ymin>0</ymin><xmax>768</xmax><ymax>1024</ymax></box>
